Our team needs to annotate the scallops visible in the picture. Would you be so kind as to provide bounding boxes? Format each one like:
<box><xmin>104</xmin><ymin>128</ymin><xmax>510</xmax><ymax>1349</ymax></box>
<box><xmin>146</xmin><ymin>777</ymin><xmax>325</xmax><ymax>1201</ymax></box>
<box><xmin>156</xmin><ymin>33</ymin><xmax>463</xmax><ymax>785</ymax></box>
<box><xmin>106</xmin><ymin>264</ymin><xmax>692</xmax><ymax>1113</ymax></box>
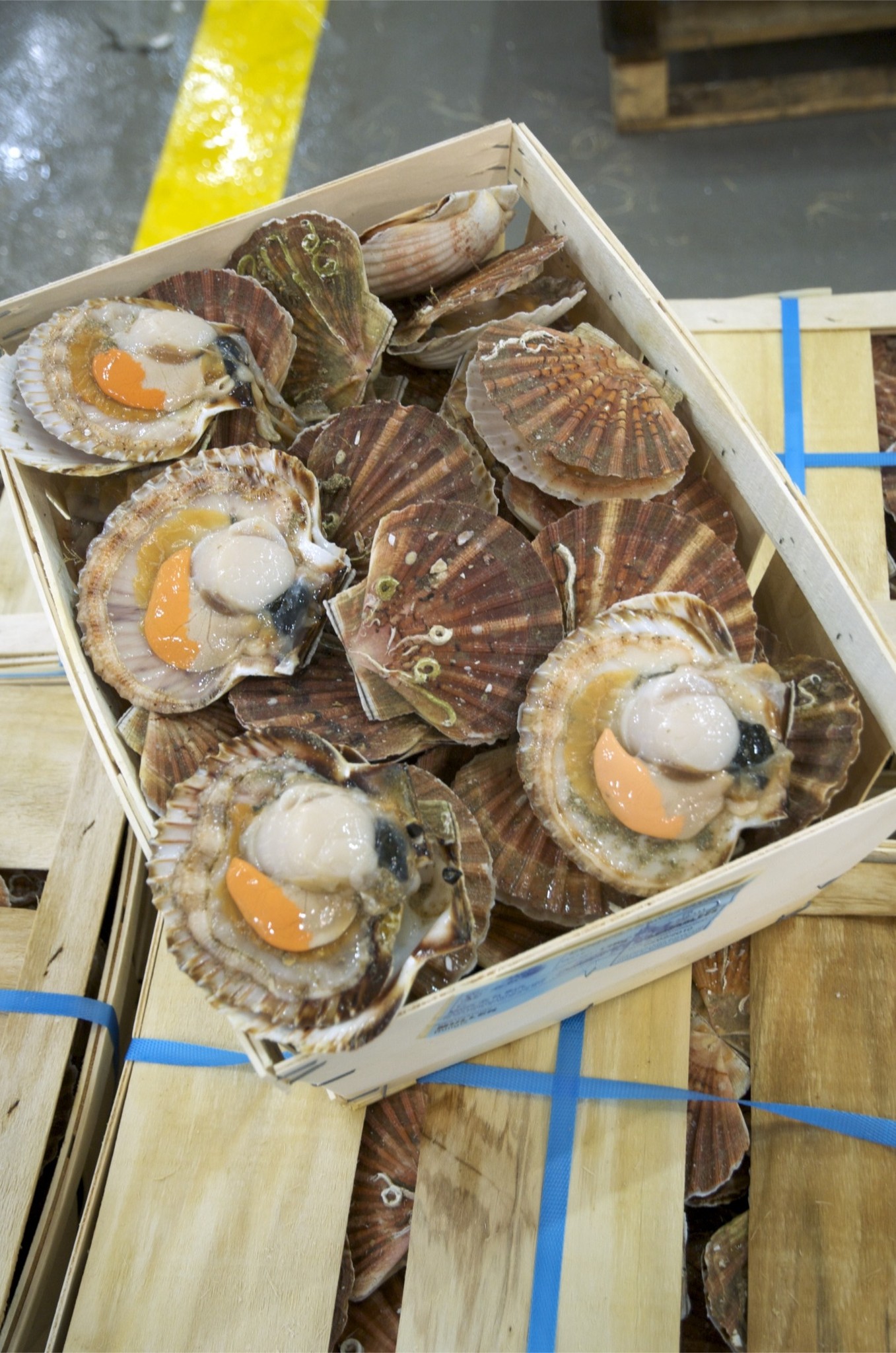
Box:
<box><xmin>3</xmin><ymin>174</ymin><xmax>867</xmax><ymax>1056</ymax></box>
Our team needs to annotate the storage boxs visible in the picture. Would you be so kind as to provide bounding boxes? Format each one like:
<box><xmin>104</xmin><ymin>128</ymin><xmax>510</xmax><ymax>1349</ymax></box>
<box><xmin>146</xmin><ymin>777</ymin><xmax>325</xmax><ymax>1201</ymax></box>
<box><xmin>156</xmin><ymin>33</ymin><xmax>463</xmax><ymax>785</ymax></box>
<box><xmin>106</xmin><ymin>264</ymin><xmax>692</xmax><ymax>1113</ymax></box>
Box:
<box><xmin>0</xmin><ymin>117</ymin><xmax>896</xmax><ymax>1353</ymax></box>
